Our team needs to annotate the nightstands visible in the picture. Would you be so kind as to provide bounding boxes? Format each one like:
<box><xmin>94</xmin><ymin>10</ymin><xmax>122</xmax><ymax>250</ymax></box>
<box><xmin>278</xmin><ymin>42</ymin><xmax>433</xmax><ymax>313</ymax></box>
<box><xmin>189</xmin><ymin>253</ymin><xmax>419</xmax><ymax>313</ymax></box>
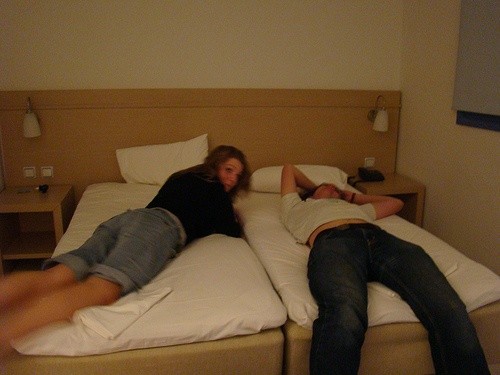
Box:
<box><xmin>347</xmin><ymin>173</ymin><xmax>426</xmax><ymax>227</ymax></box>
<box><xmin>0</xmin><ymin>185</ymin><xmax>76</xmax><ymax>279</ymax></box>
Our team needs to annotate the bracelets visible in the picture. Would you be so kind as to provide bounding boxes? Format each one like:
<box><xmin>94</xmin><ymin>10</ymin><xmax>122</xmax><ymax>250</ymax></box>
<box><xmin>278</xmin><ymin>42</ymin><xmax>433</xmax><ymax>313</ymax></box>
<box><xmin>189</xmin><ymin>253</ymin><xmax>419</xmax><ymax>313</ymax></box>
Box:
<box><xmin>350</xmin><ymin>193</ymin><xmax>356</xmax><ymax>203</ymax></box>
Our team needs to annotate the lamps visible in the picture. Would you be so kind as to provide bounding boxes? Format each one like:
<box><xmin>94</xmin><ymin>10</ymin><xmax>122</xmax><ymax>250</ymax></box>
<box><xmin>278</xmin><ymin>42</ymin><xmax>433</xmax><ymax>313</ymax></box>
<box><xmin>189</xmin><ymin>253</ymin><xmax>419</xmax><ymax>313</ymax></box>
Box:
<box><xmin>368</xmin><ymin>94</ymin><xmax>389</xmax><ymax>132</ymax></box>
<box><xmin>22</xmin><ymin>97</ymin><xmax>42</xmax><ymax>138</ymax></box>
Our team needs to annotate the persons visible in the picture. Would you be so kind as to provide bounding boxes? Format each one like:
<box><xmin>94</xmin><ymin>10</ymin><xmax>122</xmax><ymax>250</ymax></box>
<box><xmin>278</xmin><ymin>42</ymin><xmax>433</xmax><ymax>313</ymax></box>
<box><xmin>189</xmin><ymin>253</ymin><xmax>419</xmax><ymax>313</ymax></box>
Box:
<box><xmin>0</xmin><ymin>144</ymin><xmax>252</xmax><ymax>347</ymax></box>
<box><xmin>280</xmin><ymin>163</ymin><xmax>492</xmax><ymax>375</ymax></box>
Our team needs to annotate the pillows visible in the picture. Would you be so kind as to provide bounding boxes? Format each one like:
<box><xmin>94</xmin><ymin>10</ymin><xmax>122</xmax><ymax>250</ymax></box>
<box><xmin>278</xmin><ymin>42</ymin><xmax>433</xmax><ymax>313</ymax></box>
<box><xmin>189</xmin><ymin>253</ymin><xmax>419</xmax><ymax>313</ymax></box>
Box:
<box><xmin>115</xmin><ymin>133</ymin><xmax>208</xmax><ymax>188</ymax></box>
<box><xmin>250</xmin><ymin>165</ymin><xmax>349</xmax><ymax>194</ymax></box>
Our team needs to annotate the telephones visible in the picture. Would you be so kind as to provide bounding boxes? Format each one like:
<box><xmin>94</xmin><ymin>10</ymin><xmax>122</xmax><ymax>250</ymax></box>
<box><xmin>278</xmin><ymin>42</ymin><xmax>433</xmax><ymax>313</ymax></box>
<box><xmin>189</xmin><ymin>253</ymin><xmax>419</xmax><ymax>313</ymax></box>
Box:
<box><xmin>359</xmin><ymin>166</ymin><xmax>385</xmax><ymax>183</ymax></box>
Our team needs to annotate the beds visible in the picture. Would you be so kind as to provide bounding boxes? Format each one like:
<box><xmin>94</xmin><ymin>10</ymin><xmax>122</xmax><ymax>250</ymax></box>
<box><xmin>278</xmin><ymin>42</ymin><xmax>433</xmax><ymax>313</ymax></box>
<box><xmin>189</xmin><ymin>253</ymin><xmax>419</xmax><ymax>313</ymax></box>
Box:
<box><xmin>0</xmin><ymin>182</ymin><xmax>288</xmax><ymax>375</ymax></box>
<box><xmin>233</xmin><ymin>184</ymin><xmax>500</xmax><ymax>375</ymax></box>
